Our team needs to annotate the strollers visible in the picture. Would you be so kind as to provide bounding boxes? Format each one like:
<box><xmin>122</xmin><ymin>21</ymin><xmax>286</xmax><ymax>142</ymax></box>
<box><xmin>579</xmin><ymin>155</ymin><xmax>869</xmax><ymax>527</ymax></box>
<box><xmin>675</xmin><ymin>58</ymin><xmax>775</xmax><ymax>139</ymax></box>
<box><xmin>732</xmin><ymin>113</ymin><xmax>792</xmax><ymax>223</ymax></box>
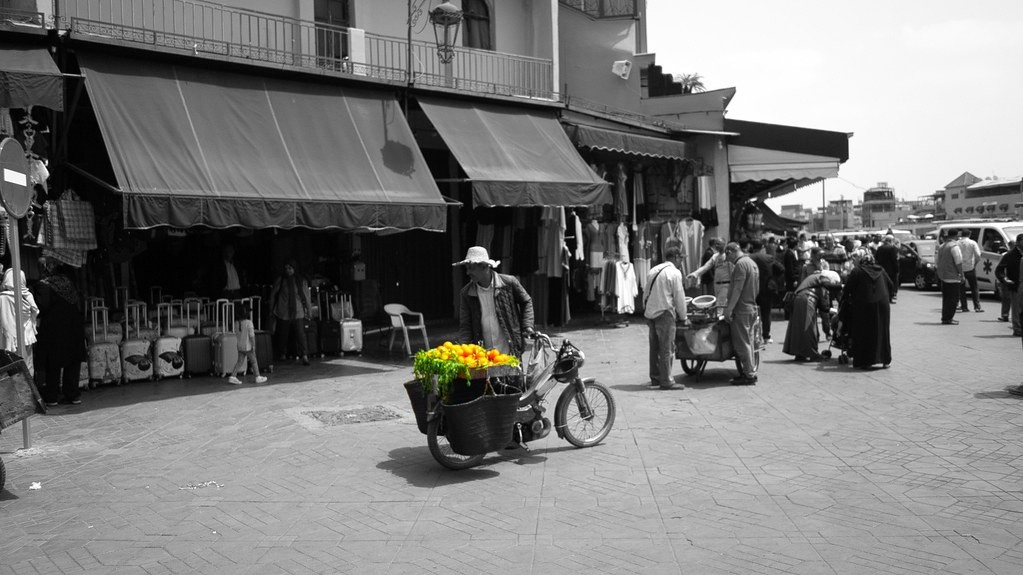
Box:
<box><xmin>820</xmin><ymin>308</ymin><xmax>850</xmax><ymax>365</ymax></box>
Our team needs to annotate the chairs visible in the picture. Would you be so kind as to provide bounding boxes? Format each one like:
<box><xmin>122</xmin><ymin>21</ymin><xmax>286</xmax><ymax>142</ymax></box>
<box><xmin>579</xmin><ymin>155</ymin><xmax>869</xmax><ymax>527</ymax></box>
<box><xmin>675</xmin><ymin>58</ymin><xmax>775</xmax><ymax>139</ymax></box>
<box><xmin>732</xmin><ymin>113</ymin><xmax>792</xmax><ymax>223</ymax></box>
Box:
<box><xmin>384</xmin><ymin>304</ymin><xmax>431</xmax><ymax>359</ymax></box>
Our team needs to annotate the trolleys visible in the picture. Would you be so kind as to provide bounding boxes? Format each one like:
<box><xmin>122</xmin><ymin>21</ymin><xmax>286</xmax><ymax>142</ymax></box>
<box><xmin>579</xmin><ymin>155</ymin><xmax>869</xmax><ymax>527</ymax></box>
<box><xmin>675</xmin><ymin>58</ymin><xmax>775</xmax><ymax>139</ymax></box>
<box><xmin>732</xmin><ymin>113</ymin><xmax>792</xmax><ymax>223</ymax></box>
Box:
<box><xmin>675</xmin><ymin>305</ymin><xmax>764</xmax><ymax>379</ymax></box>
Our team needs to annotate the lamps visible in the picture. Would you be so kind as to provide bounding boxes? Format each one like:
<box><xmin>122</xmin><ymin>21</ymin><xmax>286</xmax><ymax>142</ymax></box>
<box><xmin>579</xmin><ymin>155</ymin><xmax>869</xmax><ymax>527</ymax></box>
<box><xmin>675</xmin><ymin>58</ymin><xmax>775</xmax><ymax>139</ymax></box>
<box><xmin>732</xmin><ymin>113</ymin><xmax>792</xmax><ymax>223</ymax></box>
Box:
<box><xmin>612</xmin><ymin>59</ymin><xmax>634</xmax><ymax>79</ymax></box>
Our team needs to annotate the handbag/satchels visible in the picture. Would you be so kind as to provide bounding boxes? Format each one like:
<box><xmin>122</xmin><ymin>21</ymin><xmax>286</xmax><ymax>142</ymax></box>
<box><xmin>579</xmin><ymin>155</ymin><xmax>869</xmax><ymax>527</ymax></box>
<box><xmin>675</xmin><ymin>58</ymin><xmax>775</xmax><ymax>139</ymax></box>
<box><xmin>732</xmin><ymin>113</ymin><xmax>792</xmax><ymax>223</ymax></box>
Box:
<box><xmin>35</xmin><ymin>189</ymin><xmax>97</xmax><ymax>268</ymax></box>
<box><xmin>783</xmin><ymin>291</ymin><xmax>795</xmax><ymax>313</ymax></box>
<box><xmin>527</xmin><ymin>331</ymin><xmax>557</xmax><ymax>388</ymax></box>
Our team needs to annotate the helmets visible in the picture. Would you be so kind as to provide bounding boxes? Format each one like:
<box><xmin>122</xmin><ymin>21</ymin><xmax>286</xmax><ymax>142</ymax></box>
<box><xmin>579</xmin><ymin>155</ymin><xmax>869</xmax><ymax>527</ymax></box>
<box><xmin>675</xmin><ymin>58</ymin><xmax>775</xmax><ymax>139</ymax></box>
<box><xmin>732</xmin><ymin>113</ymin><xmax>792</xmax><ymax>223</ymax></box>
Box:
<box><xmin>553</xmin><ymin>348</ymin><xmax>585</xmax><ymax>383</ymax></box>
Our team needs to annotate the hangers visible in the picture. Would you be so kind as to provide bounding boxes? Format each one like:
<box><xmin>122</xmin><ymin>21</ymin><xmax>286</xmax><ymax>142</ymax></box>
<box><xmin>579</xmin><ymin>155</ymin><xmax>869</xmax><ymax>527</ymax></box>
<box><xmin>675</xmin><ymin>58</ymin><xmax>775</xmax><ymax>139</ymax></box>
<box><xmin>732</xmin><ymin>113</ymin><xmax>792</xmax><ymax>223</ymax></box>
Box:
<box><xmin>606</xmin><ymin>251</ymin><xmax>627</xmax><ymax>265</ymax></box>
<box><xmin>685</xmin><ymin>208</ymin><xmax>693</xmax><ymax>222</ymax></box>
<box><xmin>670</xmin><ymin>209</ymin><xmax>678</xmax><ymax>224</ymax></box>
<box><xmin>650</xmin><ymin>210</ymin><xmax>663</xmax><ymax>221</ymax></box>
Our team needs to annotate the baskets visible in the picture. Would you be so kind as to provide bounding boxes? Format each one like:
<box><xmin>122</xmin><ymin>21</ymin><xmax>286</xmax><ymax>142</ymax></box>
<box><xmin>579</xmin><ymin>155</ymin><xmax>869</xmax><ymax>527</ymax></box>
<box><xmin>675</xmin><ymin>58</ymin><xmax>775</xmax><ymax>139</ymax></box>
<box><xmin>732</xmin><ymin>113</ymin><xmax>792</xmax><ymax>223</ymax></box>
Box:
<box><xmin>441</xmin><ymin>377</ymin><xmax>523</xmax><ymax>454</ymax></box>
<box><xmin>407</xmin><ymin>378</ymin><xmax>447</xmax><ymax>436</ymax></box>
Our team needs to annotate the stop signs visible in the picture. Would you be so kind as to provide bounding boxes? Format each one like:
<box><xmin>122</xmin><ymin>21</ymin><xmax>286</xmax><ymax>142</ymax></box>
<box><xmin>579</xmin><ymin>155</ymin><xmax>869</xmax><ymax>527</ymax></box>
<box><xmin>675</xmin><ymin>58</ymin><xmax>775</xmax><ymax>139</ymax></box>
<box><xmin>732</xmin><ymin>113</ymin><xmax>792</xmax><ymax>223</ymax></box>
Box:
<box><xmin>0</xmin><ymin>138</ymin><xmax>32</xmax><ymax>219</ymax></box>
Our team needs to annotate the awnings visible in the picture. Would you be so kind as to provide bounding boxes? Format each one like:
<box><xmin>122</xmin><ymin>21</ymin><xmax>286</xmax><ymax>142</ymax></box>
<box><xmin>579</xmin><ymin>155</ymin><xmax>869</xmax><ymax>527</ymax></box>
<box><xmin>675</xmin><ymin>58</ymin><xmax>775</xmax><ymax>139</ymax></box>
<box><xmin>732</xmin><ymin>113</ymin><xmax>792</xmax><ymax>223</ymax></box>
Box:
<box><xmin>418</xmin><ymin>93</ymin><xmax>613</xmax><ymax>206</ymax></box>
<box><xmin>80</xmin><ymin>54</ymin><xmax>452</xmax><ymax>231</ymax></box>
<box><xmin>727</xmin><ymin>143</ymin><xmax>840</xmax><ymax>199</ymax></box>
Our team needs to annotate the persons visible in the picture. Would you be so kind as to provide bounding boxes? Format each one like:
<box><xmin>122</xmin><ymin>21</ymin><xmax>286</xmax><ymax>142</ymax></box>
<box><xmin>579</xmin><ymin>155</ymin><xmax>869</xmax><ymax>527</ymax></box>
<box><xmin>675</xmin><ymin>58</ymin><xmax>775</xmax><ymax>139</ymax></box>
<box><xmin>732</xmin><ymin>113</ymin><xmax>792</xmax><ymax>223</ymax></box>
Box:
<box><xmin>266</xmin><ymin>261</ymin><xmax>314</xmax><ymax>366</ymax></box>
<box><xmin>645</xmin><ymin>245</ymin><xmax>688</xmax><ymax>390</ymax></box>
<box><xmin>213</xmin><ymin>245</ymin><xmax>245</xmax><ymax>299</ymax></box>
<box><xmin>935</xmin><ymin>226</ymin><xmax>985</xmax><ymax>326</ymax></box>
<box><xmin>33</xmin><ymin>254</ymin><xmax>87</xmax><ymax>407</ymax></box>
<box><xmin>782</xmin><ymin>268</ymin><xmax>840</xmax><ymax>362</ymax></box>
<box><xmin>0</xmin><ymin>268</ymin><xmax>40</xmax><ymax>377</ymax></box>
<box><xmin>451</xmin><ymin>245</ymin><xmax>534</xmax><ymax>451</ymax></box>
<box><xmin>843</xmin><ymin>249</ymin><xmax>894</xmax><ymax>370</ymax></box>
<box><xmin>585</xmin><ymin>219</ymin><xmax>605</xmax><ymax>268</ymax></box>
<box><xmin>995</xmin><ymin>231</ymin><xmax>1023</xmax><ymax>341</ymax></box>
<box><xmin>725</xmin><ymin>241</ymin><xmax>760</xmax><ymax>388</ymax></box>
<box><xmin>225</xmin><ymin>304</ymin><xmax>269</xmax><ymax>385</ymax></box>
<box><xmin>686</xmin><ymin>231</ymin><xmax>904</xmax><ymax>345</ymax></box>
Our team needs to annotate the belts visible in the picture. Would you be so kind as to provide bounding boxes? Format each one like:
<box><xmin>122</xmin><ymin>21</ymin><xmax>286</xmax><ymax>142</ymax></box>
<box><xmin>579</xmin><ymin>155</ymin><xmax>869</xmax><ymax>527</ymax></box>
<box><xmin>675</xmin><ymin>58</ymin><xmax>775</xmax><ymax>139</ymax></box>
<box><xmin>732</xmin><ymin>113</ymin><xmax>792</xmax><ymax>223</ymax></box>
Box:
<box><xmin>716</xmin><ymin>281</ymin><xmax>730</xmax><ymax>284</ymax></box>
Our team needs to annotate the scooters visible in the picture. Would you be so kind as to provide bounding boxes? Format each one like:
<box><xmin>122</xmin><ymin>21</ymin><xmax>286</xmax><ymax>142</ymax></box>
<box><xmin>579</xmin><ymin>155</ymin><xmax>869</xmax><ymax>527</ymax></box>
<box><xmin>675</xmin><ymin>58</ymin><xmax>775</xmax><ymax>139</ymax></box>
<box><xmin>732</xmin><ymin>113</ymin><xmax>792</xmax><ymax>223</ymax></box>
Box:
<box><xmin>427</xmin><ymin>327</ymin><xmax>617</xmax><ymax>471</ymax></box>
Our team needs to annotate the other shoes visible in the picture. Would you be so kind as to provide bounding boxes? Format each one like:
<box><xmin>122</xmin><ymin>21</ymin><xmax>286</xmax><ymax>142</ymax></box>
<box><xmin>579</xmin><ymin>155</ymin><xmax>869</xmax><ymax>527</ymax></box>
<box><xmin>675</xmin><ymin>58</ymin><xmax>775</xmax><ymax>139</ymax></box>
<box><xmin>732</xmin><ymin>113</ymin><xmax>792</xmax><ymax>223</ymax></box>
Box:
<box><xmin>795</xmin><ymin>354</ymin><xmax>810</xmax><ymax>362</ymax></box>
<box><xmin>652</xmin><ymin>378</ymin><xmax>662</xmax><ymax>385</ymax></box>
<box><xmin>47</xmin><ymin>401</ymin><xmax>57</xmax><ymax>406</ymax></box>
<box><xmin>975</xmin><ymin>308</ymin><xmax>984</xmax><ymax>312</ymax></box>
<box><xmin>962</xmin><ymin>308</ymin><xmax>970</xmax><ymax>312</ymax></box>
<box><xmin>810</xmin><ymin>355</ymin><xmax>826</xmax><ymax>362</ymax></box>
<box><xmin>661</xmin><ymin>383</ymin><xmax>685</xmax><ymax>390</ymax></box>
<box><xmin>998</xmin><ymin>316</ymin><xmax>1008</xmax><ymax>321</ymax></box>
<box><xmin>1009</xmin><ymin>385</ymin><xmax>1023</xmax><ymax>396</ymax></box>
<box><xmin>942</xmin><ymin>319</ymin><xmax>958</xmax><ymax>325</ymax></box>
<box><xmin>61</xmin><ymin>399</ymin><xmax>81</xmax><ymax>404</ymax></box>
<box><xmin>729</xmin><ymin>373</ymin><xmax>757</xmax><ymax>385</ymax></box>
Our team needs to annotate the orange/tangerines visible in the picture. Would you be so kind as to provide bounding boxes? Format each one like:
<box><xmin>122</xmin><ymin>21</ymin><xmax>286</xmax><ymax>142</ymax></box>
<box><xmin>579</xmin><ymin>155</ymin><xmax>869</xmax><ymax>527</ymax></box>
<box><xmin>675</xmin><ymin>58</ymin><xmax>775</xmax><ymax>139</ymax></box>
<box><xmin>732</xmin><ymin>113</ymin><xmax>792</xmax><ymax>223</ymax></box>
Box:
<box><xmin>428</xmin><ymin>342</ymin><xmax>509</xmax><ymax>365</ymax></box>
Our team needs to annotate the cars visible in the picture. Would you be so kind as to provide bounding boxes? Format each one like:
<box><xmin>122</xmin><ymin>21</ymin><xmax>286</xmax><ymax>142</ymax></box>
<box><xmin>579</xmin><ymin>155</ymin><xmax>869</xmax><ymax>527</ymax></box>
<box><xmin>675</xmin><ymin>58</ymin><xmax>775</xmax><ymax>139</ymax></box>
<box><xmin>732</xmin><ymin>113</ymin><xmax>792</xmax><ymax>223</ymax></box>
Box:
<box><xmin>810</xmin><ymin>228</ymin><xmax>938</xmax><ymax>289</ymax></box>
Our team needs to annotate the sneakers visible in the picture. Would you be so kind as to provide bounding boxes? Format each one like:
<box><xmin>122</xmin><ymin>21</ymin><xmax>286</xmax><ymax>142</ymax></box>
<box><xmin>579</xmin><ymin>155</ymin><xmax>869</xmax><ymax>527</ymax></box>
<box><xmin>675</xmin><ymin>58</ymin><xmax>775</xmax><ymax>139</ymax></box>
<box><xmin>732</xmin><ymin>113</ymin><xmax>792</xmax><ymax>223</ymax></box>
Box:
<box><xmin>229</xmin><ymin>375</ymin><xmax>242</xmax><ymax>384</ymax></box>
<box><xmin>255</xmin><ymin>376</ymin><xmax>267</xmax><ymax>383</ymax></box>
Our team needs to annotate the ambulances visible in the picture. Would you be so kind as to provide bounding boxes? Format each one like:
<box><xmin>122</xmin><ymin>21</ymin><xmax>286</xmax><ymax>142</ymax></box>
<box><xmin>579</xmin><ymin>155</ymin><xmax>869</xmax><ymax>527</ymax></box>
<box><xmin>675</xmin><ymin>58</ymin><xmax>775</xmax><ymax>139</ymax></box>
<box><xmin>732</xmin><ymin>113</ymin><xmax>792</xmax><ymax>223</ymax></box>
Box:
<box><xmin>934</xmin><ymin>221</ymin><xmax>1023</xmax><ymax>302</ymax></box>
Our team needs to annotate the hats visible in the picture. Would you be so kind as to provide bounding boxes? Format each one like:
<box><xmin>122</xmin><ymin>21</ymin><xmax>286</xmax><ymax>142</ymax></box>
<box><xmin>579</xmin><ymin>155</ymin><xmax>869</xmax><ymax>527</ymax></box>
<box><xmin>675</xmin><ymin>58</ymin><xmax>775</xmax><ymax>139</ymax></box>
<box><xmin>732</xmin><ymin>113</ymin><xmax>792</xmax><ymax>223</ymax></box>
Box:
<box><xmin>452</xmin><ymin>246</ymin><xmax>500</xmax><ymax>269</ymax></box>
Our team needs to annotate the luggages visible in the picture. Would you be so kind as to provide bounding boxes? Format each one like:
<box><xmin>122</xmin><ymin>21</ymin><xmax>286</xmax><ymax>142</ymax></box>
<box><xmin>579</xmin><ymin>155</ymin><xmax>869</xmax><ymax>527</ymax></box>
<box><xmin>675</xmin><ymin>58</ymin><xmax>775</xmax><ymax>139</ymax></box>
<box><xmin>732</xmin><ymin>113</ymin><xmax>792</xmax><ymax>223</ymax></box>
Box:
<box><xmin>59</xmin><ymin>274</ymin><xmax>362</xmax><ymax>392</ymax></box>
